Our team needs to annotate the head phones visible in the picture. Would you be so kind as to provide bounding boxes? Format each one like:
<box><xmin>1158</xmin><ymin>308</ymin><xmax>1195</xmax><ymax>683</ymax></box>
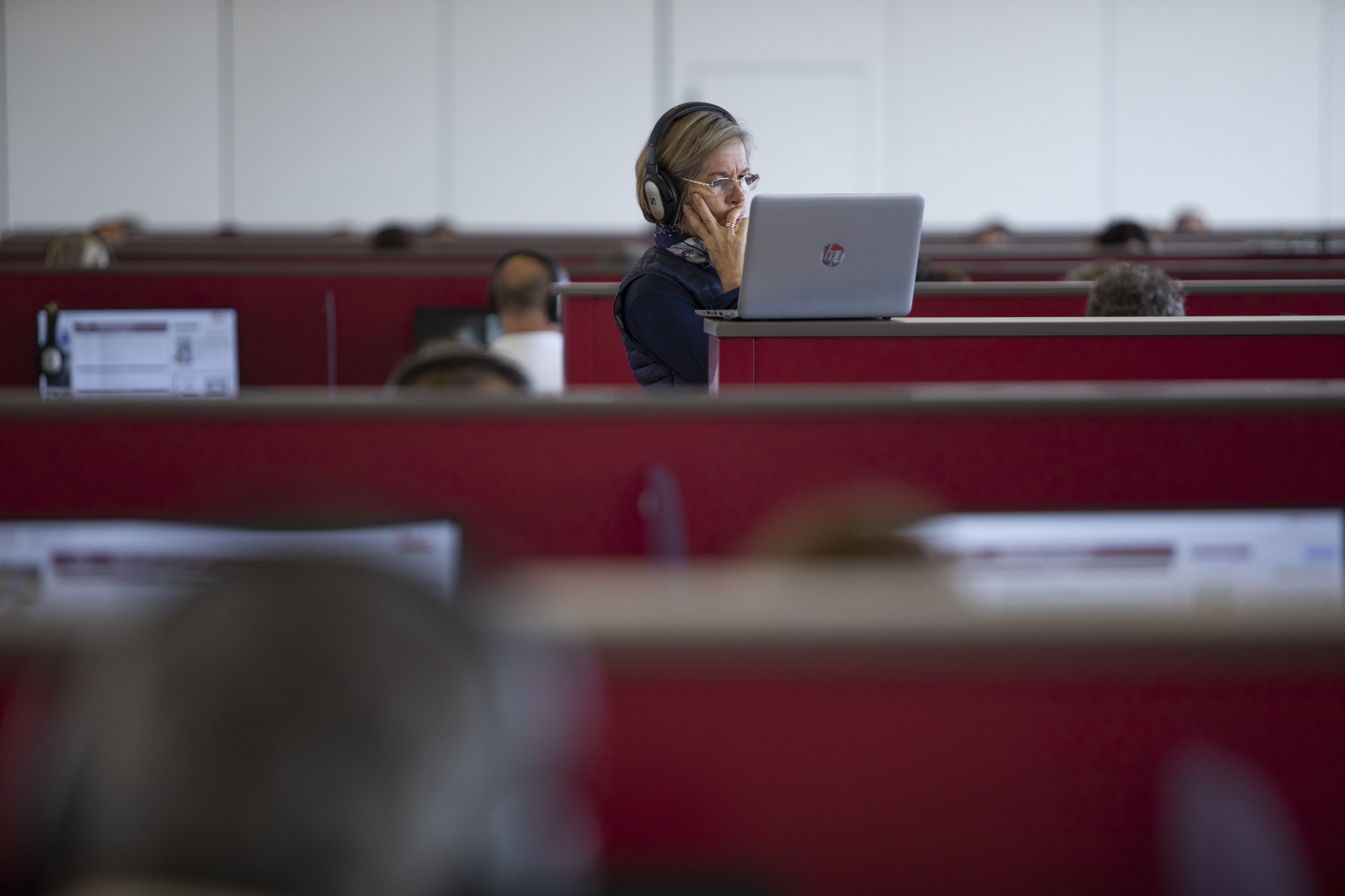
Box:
<box><xmin>643</xmin><ymin>101</ymin><xmax>738</xmax><ymax>228</ymax></box>
<box><xmin>487</xmin><ymin>248</ymin><xmax>564</xmax><ymax>324</ymax></box>
<box><xmin>36</xmin><ymin>302</ymin><xmax>64</xmax><ymax>378</ymax></box>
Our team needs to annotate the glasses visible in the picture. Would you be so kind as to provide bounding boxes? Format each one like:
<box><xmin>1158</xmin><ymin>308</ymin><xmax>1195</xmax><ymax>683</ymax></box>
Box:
<box><xmin>673</xmin><ymin>172</ymin><xmax>761</xmax><ymax>196</ymax></box>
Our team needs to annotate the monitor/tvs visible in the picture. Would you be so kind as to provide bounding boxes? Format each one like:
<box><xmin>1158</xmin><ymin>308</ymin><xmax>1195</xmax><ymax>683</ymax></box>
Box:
<box><xmin>411</xmin><ymin>305</ymin><xmax>487</xmax><ymax>355</ymax></box>
<box><xmin>896</xmin><ymin>511</ymin><xmax>1343</xmax><ymax>592</ymax></box>
<box><xmin>0</xmin><ymin>509</ymin><xmax>462</xmax><ymax>615</ymax></box>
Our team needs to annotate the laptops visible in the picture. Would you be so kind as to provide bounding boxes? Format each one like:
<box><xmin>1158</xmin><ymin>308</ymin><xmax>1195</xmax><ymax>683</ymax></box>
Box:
<box><xmin>35</xmin><ymin>306</ymin><xmax>238</xmax><ymax>400</ymax></box>
<box><xmin>693</xmin><ymin>193</ymin><xmax>924</xmax><ymax>319</ymax></box>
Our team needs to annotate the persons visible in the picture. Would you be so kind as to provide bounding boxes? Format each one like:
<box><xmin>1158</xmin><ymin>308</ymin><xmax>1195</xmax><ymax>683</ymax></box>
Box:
<box><xmin>47</xmin><ymin>196</ymin><xmax>1236</xmax><ymax>269</ymax></box>
<box><xmin>444</xmin><ymin>250</ymin><xmax>572</xmax><ymax>396</ymax></box>
<box><xmin>43</xmin><ymin>542</ymin><xmax>518</xmax><ymax>894</ymax></box>
<box><xmin>388</xmin><ymin>340</ymin><xmax>528</xmax><ymax>400</ymax></box>
<box><xmin>612</xmin><ymin>101</ymin><xmax>756</xmax><ymax>393</ymax></box>
<box><xmin>1085</xmin><ymin>259</ymin><xmax>1182</xmax><ymax>317</ymax></box>
